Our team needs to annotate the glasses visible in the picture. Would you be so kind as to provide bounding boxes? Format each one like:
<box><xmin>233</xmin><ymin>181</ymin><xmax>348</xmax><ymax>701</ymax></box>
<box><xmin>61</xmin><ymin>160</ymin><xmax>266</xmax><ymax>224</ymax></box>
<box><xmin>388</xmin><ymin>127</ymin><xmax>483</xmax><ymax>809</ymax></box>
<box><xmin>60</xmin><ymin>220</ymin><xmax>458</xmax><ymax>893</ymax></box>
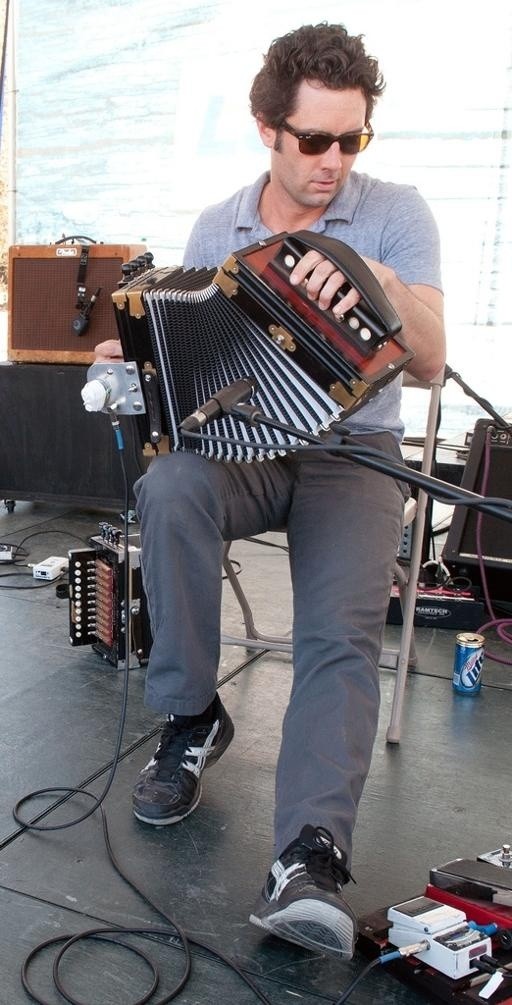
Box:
<box><xmin>279</xmin><ymin>118</ymin><xmax>373</xmax><ymax>156</ymax></box>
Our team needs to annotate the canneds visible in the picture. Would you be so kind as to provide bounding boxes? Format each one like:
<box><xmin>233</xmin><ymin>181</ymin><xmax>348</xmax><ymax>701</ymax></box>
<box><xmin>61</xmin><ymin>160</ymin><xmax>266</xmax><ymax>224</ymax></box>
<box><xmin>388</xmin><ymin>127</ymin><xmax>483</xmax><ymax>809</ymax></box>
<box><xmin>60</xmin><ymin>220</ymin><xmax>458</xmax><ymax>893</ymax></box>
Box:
<box><xmin>453</xmin><ymin>634</ymin><xmax>484</xmax><ymax>694</ymax></box>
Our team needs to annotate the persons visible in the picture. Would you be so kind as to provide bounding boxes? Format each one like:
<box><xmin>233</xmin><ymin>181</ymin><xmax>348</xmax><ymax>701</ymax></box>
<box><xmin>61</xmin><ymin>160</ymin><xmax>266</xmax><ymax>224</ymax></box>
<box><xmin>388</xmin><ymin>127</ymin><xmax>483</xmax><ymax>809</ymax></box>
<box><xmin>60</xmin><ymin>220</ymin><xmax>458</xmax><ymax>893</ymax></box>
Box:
<box><xmin>93</xmin><ymin>21</ymin><xmax>446</xmax><ymax>958</ymax></box>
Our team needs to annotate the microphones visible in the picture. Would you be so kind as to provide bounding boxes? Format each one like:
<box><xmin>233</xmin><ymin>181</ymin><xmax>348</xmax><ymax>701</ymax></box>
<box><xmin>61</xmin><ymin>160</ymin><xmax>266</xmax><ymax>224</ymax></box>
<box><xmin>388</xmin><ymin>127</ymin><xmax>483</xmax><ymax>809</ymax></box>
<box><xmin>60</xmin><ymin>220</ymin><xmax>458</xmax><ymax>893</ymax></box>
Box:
<box><xmin>70</xmin><ymin>287</ymin><xmax>102</xmax><ymax>337</ymax></box>
<box><xmin>176</xmin><ymin>374</ymin><xmax>260</xmax><ymax>436</ymax></box>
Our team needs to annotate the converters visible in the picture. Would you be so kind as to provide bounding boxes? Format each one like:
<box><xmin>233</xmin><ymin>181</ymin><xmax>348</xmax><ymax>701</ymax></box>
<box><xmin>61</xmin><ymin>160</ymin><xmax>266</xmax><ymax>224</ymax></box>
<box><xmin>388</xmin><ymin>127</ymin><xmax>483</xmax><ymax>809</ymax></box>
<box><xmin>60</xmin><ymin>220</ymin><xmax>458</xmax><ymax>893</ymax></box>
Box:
<box><xmin>32</xmin><ymin>556</ymin><xmax>70</xmax><ymax>581</ymax></box>
<box><xmin>0</xmin><ymin>545</ymin><xmax>13</xmax><ymax>561</ymax></box>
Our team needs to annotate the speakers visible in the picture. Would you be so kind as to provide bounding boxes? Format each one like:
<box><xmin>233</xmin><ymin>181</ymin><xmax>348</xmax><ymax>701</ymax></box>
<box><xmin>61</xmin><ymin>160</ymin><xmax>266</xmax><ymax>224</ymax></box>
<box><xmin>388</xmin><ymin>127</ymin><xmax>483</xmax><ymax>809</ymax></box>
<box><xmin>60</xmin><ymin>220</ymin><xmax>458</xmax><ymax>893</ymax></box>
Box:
<box><xmin>440</xmin><ymin>418</ymin><xmax>512</xmax><ymax>602</ymax></box>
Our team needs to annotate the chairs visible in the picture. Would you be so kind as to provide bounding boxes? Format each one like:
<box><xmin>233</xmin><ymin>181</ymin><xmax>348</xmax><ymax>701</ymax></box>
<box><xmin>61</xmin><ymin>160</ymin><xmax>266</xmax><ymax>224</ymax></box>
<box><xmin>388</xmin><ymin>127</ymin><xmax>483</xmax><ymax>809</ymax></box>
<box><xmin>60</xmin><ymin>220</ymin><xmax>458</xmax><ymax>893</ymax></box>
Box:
<box><xmin>217</xmin><ymin>363</ymin><xmax>448</xmax><ymax>745</ymax></box>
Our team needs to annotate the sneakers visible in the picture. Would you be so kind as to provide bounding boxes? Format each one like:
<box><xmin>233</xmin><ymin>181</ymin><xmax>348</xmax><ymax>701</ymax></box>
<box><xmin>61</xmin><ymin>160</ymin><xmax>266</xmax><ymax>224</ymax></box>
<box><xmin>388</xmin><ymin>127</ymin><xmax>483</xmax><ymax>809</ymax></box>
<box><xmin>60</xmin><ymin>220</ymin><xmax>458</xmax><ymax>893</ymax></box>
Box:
<box><xmin>133</xmin><ymin>690</ymin><xmax>234</xmax><ymax>826</ymax></box>
<box><xmin>248</xmin><ymin>823</ymin><xmax>357</xmax><ymax>961</ymax></box>
